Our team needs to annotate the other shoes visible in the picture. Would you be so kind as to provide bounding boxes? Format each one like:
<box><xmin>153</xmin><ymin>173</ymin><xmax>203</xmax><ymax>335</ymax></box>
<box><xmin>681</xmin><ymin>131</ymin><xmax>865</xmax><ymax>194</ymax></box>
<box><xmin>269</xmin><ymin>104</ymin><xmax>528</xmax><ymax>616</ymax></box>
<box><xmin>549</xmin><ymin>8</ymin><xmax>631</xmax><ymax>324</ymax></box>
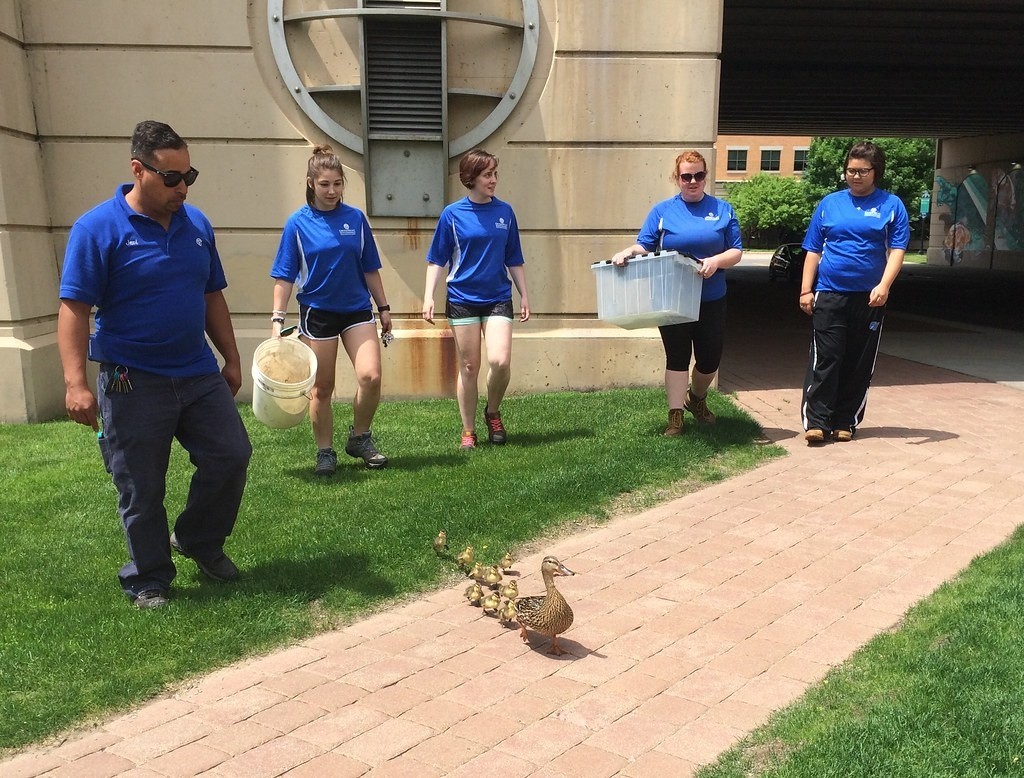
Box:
<box><xmin>833</xmin><ymin>429</ymin><xmax>851</xmax><ymax>440</ymax></box>
<box><xmin>805</xmin><ymin>428</ymin><xmax>830</xmax><ymax>441</ymax></box>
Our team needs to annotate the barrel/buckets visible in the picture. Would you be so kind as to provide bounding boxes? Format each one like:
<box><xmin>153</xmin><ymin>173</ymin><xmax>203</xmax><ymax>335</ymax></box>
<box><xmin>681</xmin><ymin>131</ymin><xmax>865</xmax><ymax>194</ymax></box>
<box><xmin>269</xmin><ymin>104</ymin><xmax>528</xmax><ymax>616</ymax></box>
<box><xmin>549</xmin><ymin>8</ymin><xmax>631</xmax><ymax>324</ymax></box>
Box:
<box><xmin>251</xmin><ymin>337</ymin><xmax>318</xmax><ymax>428</ymax></box>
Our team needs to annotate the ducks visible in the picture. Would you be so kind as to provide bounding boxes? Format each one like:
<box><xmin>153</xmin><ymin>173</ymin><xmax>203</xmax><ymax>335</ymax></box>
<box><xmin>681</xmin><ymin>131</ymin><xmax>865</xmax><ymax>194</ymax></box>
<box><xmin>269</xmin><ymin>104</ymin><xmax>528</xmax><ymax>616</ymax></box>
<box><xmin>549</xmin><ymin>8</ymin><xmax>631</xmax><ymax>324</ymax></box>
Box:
<box><xmin>432</xmin><ymin>531</ymin><xmax>577</xmax><ymax>659</ymax></box>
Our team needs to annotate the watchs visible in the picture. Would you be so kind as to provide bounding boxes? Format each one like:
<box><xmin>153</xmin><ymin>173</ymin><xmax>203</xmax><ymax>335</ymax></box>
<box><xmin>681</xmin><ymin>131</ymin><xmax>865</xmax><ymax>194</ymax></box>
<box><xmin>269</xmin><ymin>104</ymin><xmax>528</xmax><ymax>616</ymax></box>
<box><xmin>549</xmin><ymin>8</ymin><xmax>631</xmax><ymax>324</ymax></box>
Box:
<box><xmin>378</xmin><ymin>305</ymin><xmax>390</xmax><ymax>313</ymax></box>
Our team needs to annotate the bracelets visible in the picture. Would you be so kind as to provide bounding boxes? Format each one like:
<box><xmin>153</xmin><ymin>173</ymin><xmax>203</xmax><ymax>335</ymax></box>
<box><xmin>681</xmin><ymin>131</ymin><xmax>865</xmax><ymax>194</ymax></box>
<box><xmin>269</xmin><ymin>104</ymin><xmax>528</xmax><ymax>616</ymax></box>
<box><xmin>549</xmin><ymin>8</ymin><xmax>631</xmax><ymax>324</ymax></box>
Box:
<box><xmin>271</xmin><ymin>317</ymin><xmax>285</xmax><ymax>324</ymax></box>
<box><xmin>272</xmin><ymin>310</ymin><xmax>287</xmax><ymax>315</ymax></box>
<box><xmin>800</xmin><ymin>291</ymin><xmax>812</xmax><ymax>297</ymax></box>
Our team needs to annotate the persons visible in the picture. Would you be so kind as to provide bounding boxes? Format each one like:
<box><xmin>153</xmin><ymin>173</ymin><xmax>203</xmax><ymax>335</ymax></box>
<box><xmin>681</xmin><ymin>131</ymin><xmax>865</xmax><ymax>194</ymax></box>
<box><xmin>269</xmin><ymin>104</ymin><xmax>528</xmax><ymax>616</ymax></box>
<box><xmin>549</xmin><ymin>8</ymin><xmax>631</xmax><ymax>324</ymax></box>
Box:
<box><xmin>270</xmin><ymin>145</ymin><xmax>391</xmax><ymax>476</ymax></box>
<box><xmin>798</xmin><ymin>143</ymin><xmax>910</xmax><ymax>442</ymax></box>
<box><xmin>56</xmin><ymin>120</ymin><xmax>252</xmax><ymax>612</ymax></box>
<box><xmin>611</xmin><ymin>149</ymin><xmax>742</xmax><ymax>433</ymax></box>
<box><xmin>422</xmin><ymin>147</ymin><xmax>529</xmax><ymax>450</ymax></box>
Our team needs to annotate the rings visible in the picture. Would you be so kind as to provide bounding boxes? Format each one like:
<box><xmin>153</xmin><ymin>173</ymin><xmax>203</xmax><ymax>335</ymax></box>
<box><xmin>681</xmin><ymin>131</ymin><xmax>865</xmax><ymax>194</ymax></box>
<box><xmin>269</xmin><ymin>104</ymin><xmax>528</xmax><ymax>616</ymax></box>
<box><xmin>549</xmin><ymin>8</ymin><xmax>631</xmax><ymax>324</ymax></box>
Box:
<box><xmin>800</xmin><ymin>305</ymin><xmax>803</xmax><ymax>308</ymax></box>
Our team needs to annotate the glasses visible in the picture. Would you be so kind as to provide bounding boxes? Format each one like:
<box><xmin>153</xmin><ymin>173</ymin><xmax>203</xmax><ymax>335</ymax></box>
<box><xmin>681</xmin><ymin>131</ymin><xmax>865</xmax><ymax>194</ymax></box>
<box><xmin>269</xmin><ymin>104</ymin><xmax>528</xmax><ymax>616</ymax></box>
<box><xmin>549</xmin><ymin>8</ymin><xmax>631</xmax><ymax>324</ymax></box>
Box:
<box><xmin>677</xmin><ymin>171</ymin><xmax>706</xmax><ymax>183</ymax></box>
<box><xmin>130</xmin><ymin>158</ymin><xmax>199</xmax><ymax>187</ymax></box>
<box><xmin>844</xmin><ymin>166</ymin><xmax>874</xmax><ymax>176</ymax></box>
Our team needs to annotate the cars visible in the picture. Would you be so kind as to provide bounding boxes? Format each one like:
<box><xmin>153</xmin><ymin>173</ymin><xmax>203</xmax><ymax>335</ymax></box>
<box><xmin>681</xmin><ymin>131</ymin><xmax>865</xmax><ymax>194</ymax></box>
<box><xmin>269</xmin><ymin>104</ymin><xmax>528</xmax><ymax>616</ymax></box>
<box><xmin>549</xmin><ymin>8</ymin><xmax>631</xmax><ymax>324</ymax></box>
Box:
<box><xmin>768</xmin><ymin>242</ymin><xmax>819</xmax><ymax>293</ymax></box>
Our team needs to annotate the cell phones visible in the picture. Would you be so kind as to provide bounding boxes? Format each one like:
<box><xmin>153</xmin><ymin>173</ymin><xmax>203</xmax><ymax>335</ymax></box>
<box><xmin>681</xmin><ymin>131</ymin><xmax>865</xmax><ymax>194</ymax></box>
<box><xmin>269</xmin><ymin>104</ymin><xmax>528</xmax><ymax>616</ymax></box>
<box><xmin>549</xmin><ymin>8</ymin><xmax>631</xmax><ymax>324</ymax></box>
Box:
<box><xmin>281</xmin><ymin>326</ymin><xmax>297</xmax><ymax>337</ymax></box>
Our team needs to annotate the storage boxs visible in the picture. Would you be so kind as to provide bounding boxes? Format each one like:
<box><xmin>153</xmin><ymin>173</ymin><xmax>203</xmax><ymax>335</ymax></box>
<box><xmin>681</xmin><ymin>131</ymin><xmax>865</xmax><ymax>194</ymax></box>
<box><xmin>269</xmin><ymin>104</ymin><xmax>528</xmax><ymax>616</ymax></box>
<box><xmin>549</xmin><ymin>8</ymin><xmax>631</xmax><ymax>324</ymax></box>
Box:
<box><xmin>590</xmin><ymin>253</ymin><xmax>700</xmax><ymax>330</ymax></box>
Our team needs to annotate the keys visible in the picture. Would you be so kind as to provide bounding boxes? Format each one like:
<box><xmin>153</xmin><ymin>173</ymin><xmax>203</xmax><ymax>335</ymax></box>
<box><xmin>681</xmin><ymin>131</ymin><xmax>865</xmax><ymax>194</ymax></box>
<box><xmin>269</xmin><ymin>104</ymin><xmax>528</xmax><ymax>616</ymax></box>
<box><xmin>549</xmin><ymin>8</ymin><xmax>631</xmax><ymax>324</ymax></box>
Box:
<box><xmin>111</xmin><ymin>371</ymin><xmax>133</xmax><ymax>394</ymax></box>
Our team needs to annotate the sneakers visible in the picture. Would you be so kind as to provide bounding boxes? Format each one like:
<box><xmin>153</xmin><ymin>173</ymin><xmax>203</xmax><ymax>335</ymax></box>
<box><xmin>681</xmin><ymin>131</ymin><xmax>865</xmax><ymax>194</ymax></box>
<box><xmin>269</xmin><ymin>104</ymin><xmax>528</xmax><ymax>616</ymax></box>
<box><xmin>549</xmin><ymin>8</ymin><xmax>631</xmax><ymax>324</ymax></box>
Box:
<box><xmin>483</xmin><ymin>405</ymin><xmax>506</xmax><ymax>443</ymax></box>
<box><xmin>133</xmin><ymin>591</ymin><xmax>167</xmax><ymax>608</ymax></box>
<box><xmin>315</xmin><ymin>449</ymin><xmax>337</xmax><ymax>472</ymax></box>
<box><xmin>684</xmin><ymin>389</ymin><xmax>716</xmax><ymax>424</ymax></box>
<box><xmin>664</xmin><ymin>410</ymin><xmax>685</xmax><ymax>435</ymax></box>
<box><xmin>170</xmin><ymin>532</ymin><xmax>239</xmax><ymax>581</ymax></box>
<box><xmin>345</xmin><ymin>425</ymin><xmax>388</xmax><ymax>468</ymax></box>
<box><xmin>460</xmin><ymin>432</ymin><xmax>477</xmax><ymax>449</ymax></box>
